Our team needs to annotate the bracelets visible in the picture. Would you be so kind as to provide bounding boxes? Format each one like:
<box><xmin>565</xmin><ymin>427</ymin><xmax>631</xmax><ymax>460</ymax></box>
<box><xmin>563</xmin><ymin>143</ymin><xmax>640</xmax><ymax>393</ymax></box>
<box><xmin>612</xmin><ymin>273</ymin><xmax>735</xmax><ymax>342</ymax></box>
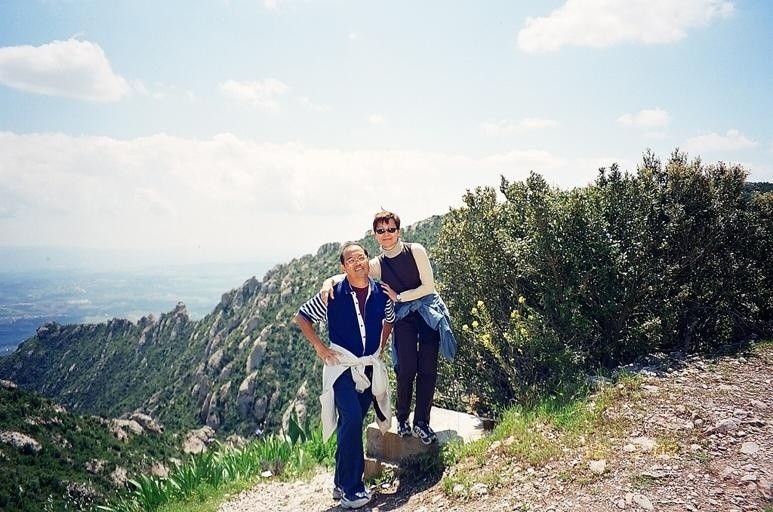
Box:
<box><xmin>327</xmin><ymin>279</ymin><xmax>334</xmax><ymax>285</ymax></box>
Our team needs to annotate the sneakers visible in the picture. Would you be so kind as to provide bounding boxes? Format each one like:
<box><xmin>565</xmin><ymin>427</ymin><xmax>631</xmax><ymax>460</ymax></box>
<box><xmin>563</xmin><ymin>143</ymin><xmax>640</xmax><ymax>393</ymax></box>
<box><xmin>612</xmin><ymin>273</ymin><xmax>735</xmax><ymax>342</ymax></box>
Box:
<box><xmin>396</xmin><ymin>418</ymin><xmax>413</xmax><ymax>440</ymax></box>
<box><xmin>332</xmin><ymin>484</ymin><xmax>372</xmax><ymax>508</ymax></box>
<box><xmin>411</xmin><ymin>419</ymin><xmax>437</xmax><ymax>445</ymax></box>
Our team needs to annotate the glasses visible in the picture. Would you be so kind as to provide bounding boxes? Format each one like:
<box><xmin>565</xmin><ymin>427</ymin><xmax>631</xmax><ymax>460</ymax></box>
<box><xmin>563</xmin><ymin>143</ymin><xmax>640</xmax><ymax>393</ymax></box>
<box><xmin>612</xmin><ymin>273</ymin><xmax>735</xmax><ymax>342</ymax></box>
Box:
<box><xmin>343</xmin><ymin>255</ymin><xmax>368</xmax><ymax>265</ymax></box>
<box><xmin>374</xmin><ymin>227</ymin><xmax>397</xmax><ymax>235</ymax></box>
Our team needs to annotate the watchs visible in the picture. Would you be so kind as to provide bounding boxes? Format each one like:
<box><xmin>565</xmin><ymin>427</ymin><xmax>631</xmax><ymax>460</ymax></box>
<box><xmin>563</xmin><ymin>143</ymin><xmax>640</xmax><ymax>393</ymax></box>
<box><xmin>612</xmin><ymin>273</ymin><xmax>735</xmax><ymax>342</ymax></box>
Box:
<box><xmin>396</xmin><ymin>293</ymin><xmax>400</xmax><ymax>302</ymax></box>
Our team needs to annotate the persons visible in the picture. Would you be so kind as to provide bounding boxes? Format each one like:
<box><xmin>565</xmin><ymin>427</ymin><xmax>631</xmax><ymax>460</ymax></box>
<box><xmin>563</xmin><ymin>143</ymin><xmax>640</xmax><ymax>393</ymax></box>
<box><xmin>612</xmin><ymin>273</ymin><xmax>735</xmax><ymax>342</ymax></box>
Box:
<box><xmin>295</xmin><ymin>244</ymin><xmax>396</xmax><ymax>510</ymax></box>
<box><xmin>321</xmin><ymin>207</ymin><xmax>441</xmax><ymax>445</ymax></box>
